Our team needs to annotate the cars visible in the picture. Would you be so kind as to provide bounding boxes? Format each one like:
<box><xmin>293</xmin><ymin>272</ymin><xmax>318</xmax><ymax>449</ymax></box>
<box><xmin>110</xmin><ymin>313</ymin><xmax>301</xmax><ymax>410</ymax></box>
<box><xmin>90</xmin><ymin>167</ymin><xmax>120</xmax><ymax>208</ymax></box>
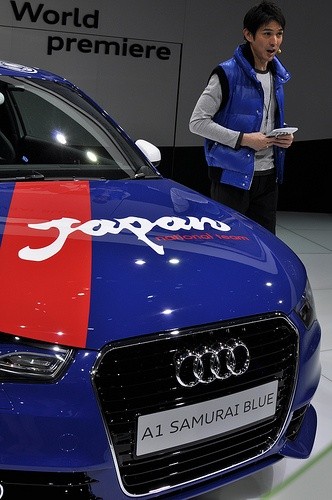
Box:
<box><xmin>0</xmin><ymin>59</ymin><xmax>322</xmax><ymax>500</ymax></box>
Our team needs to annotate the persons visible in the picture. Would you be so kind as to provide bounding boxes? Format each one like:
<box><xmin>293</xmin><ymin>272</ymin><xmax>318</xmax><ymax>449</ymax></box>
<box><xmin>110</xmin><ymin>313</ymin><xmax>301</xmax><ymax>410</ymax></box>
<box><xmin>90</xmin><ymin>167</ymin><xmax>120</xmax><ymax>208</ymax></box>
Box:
<box><xmin>189</xmin><ymin>1</ymin><xmax>298</xmax><ymax>236</ymax></box>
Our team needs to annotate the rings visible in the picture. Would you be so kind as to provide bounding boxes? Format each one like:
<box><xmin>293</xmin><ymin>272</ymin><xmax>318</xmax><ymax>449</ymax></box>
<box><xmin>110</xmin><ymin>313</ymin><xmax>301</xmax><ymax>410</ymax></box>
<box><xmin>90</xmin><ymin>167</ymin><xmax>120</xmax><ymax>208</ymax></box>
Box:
<box><xmin>266</xmin><ymin>145</ymin><xmax>269</xmax><ymax>149</ymax></box>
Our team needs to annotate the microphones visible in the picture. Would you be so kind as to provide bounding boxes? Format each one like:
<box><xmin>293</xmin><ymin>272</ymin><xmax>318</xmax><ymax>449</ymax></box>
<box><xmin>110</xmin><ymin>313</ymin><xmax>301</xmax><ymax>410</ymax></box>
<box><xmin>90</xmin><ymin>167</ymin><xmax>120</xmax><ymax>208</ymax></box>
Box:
<box><xmin>277</xmin><ymin>49</ymin><xmax>281</xmax><ymax>54</ymax></box>
